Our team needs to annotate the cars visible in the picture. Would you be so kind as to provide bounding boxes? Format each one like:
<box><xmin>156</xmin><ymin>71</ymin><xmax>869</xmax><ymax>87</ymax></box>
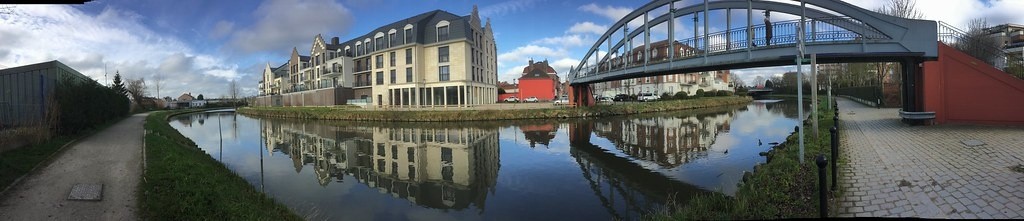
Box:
<box><xmin>562</xmin><ymin>95</ymin><xmax>568</xmax><ymax>100</ymax></box>
<box><xmin>637</xmin><ymin>92</ymin><xmax>661</xmax><ymax>101</ymax></box>
<box><xmin>615</xmin><ymin>94</ymin><xmax>634</xmax><ymax>101</ymax></box>
<box><xmin>601</xmin><ymin>97</ymin><xmax>614</xmax><ymax>102</ymax></box>
<box><xmin>555</xmin><ymin>98</ymin><xmax>569</xmax><ymax>105</ymax></box>
<box><xmin>523</xmin><ymin>96</ymin><xmax>538</xmax><ymax>103</ymax></box>
<box><xmin>503</xmin><ymin>96</ymin><xmax>519</xmax><ymax>103</ymax></box>
